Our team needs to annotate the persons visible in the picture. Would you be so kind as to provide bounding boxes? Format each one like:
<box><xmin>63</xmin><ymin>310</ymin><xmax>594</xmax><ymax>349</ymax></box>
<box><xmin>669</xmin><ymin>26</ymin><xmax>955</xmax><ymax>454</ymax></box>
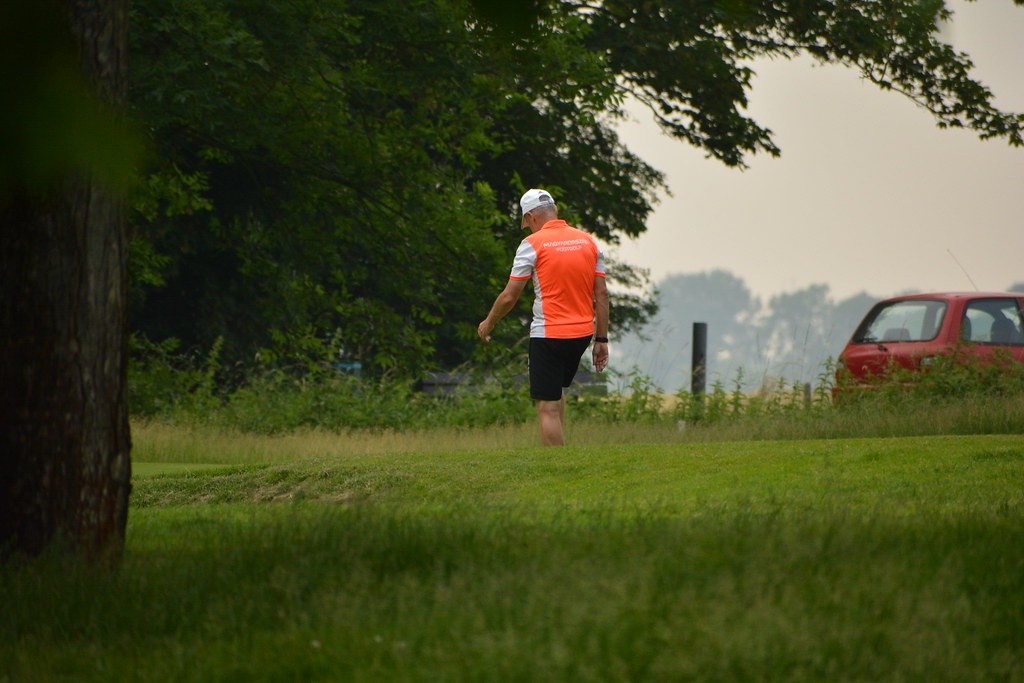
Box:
<box><xmin>478</xmin><ymin>189</ymin><xmax>610</xmax><ymax>447</ymax></box>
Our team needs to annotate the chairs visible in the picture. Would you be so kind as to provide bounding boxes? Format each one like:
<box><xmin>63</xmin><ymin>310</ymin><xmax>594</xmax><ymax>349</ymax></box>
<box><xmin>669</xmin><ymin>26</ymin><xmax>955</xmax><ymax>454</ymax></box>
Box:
<box><xmin>961</xmin><ymin>316</ymin><xmax>971</xmax><ymax>341</ymax></box>
<box><xmin>991</xmin><ymin>319</ymin><xmax>1019</xmax><ymax>343</ymax></box>
<box><xmin>884</xmin><ymin>328</ymin><xmax>911</xmax><ymax>340</ymax></box>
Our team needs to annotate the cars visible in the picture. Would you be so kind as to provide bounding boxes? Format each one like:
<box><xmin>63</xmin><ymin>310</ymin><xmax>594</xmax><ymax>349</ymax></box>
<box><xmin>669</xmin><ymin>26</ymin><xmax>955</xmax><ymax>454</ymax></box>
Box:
<box><xmin>832</xmin><ymin>291</ymin><xmax>1024</xmax><ymax>410</ymax></box>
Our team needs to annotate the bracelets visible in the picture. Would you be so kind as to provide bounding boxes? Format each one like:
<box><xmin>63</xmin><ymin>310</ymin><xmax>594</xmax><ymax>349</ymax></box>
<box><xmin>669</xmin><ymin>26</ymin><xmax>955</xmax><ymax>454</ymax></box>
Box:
<box><xmin>594</xmin><ymin>336</ymin><xmax>609</xmax><ymax>343</ymax></box>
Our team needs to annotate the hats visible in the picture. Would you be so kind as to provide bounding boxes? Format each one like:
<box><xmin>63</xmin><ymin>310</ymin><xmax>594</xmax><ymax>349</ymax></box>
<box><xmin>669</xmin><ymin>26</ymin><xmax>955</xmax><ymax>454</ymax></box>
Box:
<box><xmin>519</xmin><ymin>187</ymin><xmax>555</xmax><ymax>231</ymax></box>
